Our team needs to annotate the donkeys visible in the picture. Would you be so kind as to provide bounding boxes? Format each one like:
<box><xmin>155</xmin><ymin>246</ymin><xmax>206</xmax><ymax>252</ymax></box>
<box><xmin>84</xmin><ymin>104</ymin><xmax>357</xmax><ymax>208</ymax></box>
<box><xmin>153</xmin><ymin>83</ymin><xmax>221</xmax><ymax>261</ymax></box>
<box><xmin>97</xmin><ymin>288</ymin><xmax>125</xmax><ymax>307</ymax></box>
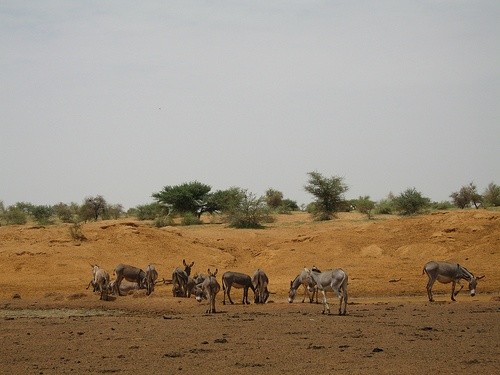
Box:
<box><xmin>288</xmin><ymin>271</ymin><xmax>318</xmax><ymax>303</ymax></box>
<box><xmin>251</xmin><ymin>269</ymin><xmax>276</xmax><ymax>304</ymax></box>
<box><xmin>86</xmin><ymin>262</ymin><xmax>146</xmax><ymax>301</ymax></box>
<box><xmin>423</xmin><ymin>261</ymin><xmax>485</xmax><ymax>302</ymax></box>
<box><xmin>304</xmin><ymin>265</ymin><xmax>348</xmax><ymax>315</ymax></box>
<box><xmin>146</xmin><ymin>265</ymin><xmax>158</xmax><ymax>296</ymax></box>
<box><xmin>222</xmin><ymin>271</ymin><xmax>255</xmax><ymax>305</ymax></box>
<box><xmin>172</xmin><ymin>259</ymin><xmax>220</xmax><ymax>314</ymax></box>
